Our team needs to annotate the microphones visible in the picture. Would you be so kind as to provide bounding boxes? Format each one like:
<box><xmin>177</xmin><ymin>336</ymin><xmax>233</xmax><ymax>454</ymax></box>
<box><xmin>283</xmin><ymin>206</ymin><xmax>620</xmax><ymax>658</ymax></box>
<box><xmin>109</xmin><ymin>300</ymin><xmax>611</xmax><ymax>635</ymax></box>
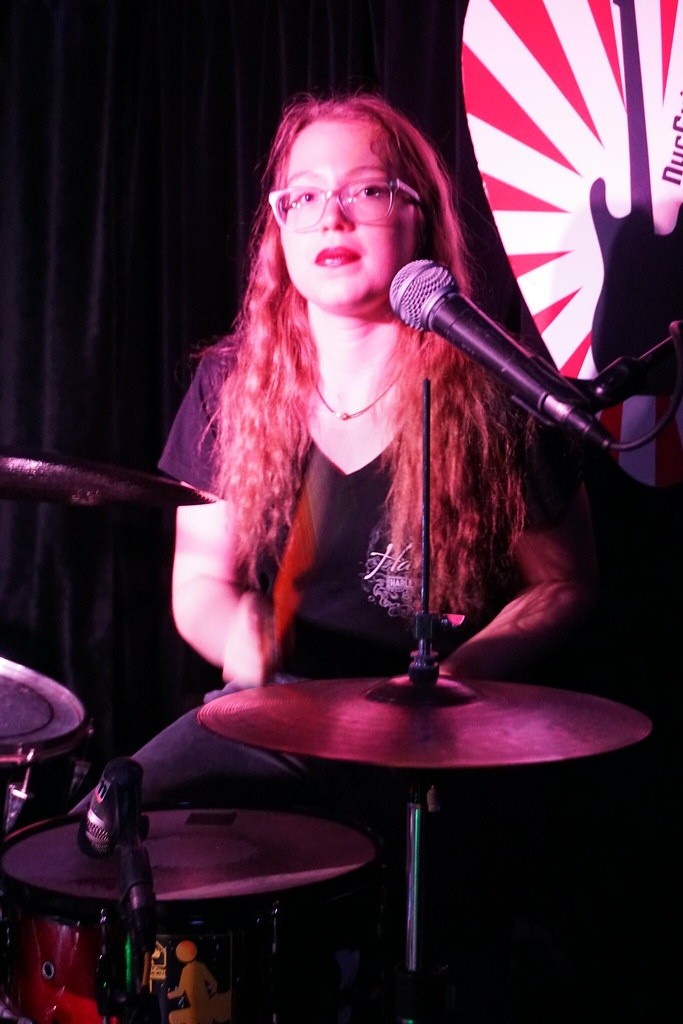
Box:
<box><xmin>389</xmin><ymin>260</ymin><xmax>614</xmax><ymax>451</ymax></box>
<box><xmin>77</xmin><ymin>757</ymin><xmax>144</xmax><ymax>858</ymax></box>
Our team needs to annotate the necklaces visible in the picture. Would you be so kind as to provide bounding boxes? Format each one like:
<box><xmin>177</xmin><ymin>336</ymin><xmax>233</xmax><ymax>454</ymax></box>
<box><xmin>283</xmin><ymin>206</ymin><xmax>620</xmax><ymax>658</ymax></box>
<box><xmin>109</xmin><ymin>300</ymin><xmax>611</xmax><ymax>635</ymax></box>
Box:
<box><xmin>301</xmin><ymin>366</ymin><xmax>399</xmax><ymax>424</ymax></box>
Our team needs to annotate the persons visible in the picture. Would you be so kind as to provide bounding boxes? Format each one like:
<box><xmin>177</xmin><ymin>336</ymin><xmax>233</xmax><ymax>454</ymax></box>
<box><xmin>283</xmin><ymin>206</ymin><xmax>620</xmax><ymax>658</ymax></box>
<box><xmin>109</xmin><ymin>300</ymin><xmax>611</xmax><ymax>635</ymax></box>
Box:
<box><xmin>69</xmin><ymin>90</ymin><xmax>589</xmax><ymax>1024</ymax></box>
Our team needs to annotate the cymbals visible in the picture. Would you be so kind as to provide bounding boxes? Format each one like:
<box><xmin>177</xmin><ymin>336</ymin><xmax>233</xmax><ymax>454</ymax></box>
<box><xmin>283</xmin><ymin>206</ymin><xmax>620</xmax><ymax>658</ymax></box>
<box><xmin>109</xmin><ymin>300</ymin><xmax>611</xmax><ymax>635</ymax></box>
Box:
<box><xmin>0</xmin><ymin>450</ymin><xmax>220</xmax><ymax>508</ymax></box>
<box><xmin>197</xmin><ymin>662</ymin><xmax>653</xmax><ymax>769</ymax></box>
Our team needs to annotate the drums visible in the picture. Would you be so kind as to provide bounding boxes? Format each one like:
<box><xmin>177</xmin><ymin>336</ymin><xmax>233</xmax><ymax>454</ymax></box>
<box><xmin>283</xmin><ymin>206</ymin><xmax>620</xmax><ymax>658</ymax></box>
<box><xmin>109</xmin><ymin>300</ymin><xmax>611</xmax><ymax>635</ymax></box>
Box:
<box><xmin>0</xmin><ymin>808</ymin><xmax>383</xmax><ymax>1024</ymax></box>
<box><xmin>162</xmin><ymin>910</ymin><xmax>170</xmax><ymax>912</ymax></box>
<box><xmin>0</xmin><ymin>656</ymin><xmax>95</xmax><ymax>838</ymax></box>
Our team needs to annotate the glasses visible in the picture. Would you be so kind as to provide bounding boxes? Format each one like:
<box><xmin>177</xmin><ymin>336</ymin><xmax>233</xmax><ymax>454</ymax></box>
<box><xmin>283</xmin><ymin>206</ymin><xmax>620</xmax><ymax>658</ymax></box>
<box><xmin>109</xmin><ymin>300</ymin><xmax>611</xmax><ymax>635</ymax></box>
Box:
<box><xmin>269</xmin><ymin>178</ymin><xmax>423</xmax><ymax>233</ymax></box>
